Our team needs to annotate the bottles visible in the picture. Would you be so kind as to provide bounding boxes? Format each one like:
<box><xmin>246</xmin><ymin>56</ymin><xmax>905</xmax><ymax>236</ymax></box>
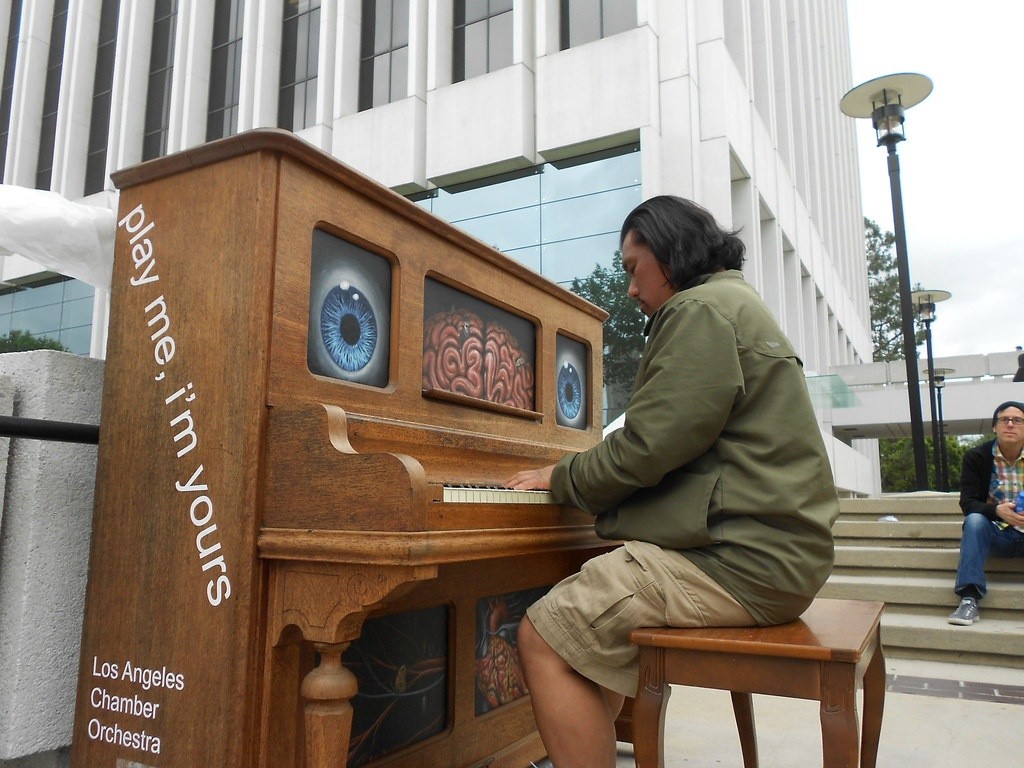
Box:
<box><xmin>1012</xmin><ymin>489</ymin><xmax>1024</xmax><ymax>533</ymax></box>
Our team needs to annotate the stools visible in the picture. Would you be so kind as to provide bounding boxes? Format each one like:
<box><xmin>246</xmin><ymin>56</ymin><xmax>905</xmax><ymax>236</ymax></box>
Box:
<box><xmin>631</xmin><ymin>596</ymin><xmax>888</xmax><ymax>768</ymax></box>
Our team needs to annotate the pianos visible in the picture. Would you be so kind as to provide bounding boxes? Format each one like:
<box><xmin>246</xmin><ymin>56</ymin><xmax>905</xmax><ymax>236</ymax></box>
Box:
<box><xmin>70</xmin><ymin>127</ymin><xmax>636</xmax><ymax>768</ymax></box>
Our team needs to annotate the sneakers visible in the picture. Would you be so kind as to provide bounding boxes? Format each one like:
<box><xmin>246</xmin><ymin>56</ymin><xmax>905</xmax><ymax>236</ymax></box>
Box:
<box><xmin>948</xmin><ymin>596</ymin><xmax>980</xmax><ymax>625</ymax></box>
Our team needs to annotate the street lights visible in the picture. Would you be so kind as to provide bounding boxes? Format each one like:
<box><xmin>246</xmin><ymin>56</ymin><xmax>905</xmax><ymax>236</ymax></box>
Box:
<box><xmin>911</xmin><ymin>290</ymin><xmax>952</xmax><ymax>491</ymax></box>
<box><xmin>838</xmin><ymin>72</ymin><xmax>934</xmax><ymax>492</ymax></box>
<box><xmin>922</xmin><ymin>368</ymin><xmax>955</xmax><ymax>492</ymax></box>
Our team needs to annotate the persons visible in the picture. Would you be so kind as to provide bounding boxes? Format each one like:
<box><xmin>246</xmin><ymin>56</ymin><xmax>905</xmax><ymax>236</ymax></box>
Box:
<box><xmin>947</xmin><ymin>401</ymin><xmax>1024</xmax><ymax>626</ymax></box>
<box><xmin>503</xmin><ymin>195</ymin><xmax>840</xmax><ymax>768</ymax></box>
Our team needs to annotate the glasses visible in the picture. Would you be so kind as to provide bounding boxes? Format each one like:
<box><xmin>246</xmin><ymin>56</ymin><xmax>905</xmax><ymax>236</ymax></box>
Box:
<box><xmin>995</xmin><ymin>417</ymin><xmax>1024</xmax><ymax>425</ymax></box>
